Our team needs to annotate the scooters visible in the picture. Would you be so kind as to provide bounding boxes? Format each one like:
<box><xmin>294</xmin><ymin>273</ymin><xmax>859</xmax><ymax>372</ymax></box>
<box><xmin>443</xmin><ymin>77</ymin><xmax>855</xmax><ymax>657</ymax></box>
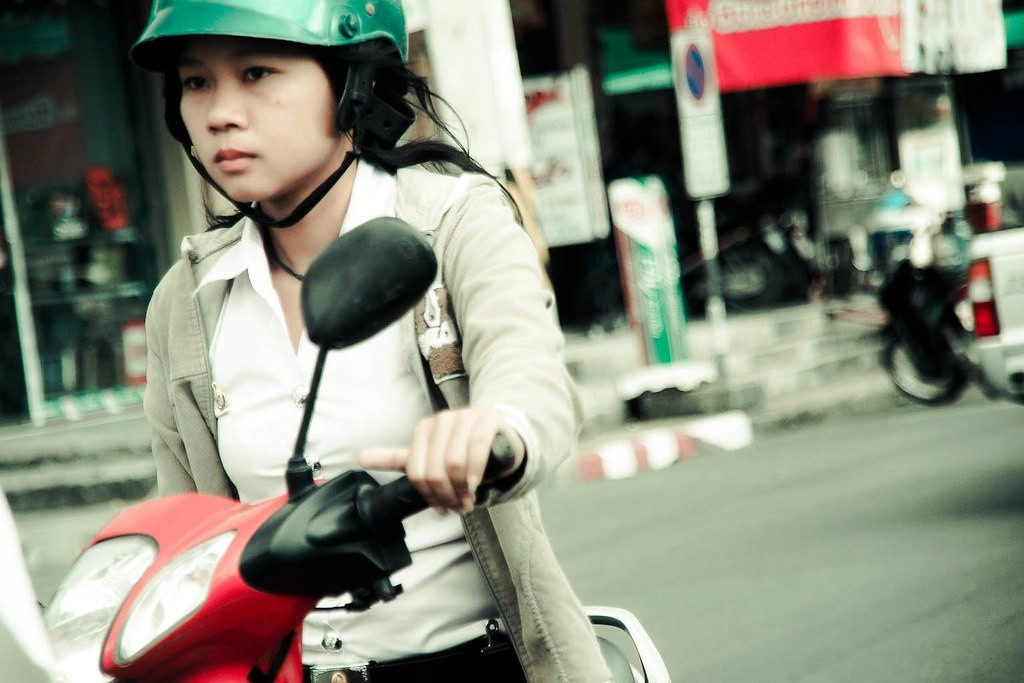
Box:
<box><xmin>36</xmin><ymin>215</ymin><xmax>674</xmax><ymax>683</ymax></box>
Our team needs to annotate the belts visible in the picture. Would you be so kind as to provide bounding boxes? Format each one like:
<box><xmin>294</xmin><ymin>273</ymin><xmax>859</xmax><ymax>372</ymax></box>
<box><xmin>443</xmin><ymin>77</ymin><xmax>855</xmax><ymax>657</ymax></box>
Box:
<box><xmin>302</xmin><ymin>648</ymin><xmax>528</xmax><ymax>683</ymax></box>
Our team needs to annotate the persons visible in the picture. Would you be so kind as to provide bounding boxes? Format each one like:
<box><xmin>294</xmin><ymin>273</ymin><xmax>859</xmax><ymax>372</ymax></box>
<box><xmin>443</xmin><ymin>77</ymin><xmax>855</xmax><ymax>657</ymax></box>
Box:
<box><xmin>130</xmin><ymin>0</ymin><xmax>614</xmax><ymax>683</ymax></box>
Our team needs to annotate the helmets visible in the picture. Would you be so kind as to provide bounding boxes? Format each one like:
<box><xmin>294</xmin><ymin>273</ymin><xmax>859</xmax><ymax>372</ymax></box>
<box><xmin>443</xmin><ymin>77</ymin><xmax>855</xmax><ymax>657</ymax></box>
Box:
<box><xmin>131</xmin><ymin>0</ymin><xmax>409</xmax><ymax>75</ymax></box>
